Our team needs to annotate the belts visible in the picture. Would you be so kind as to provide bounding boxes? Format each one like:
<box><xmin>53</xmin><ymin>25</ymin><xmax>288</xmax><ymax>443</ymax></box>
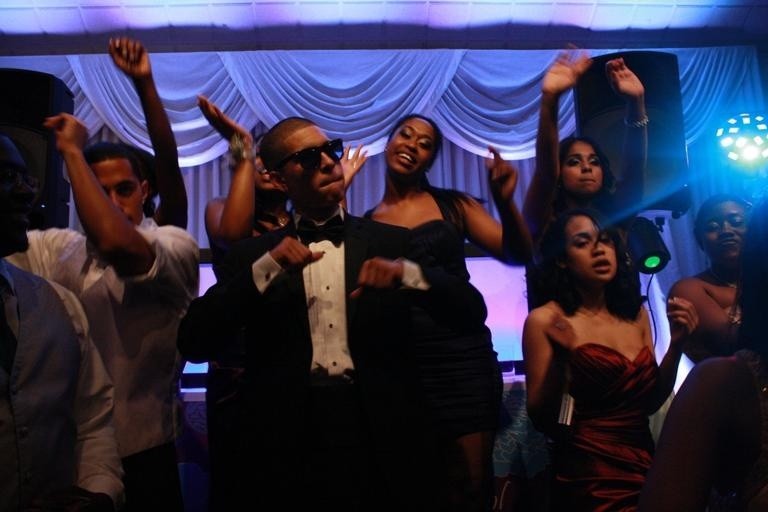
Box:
<box><xmin>304</xmin><ymin>372</ymin><xmax>361</xmax><ymax>387</ymax></box>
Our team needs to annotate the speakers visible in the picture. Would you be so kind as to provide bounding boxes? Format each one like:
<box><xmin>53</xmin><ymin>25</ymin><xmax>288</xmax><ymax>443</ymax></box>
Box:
<box><xmin>573</xmin><ymin>50</ymin><xmax>692</xmax><ymax>211</ymax></box>
<box><xmin>0</xmin><ymin>68</ymin><xmax>75</xmax><ymax>229</ymax></box>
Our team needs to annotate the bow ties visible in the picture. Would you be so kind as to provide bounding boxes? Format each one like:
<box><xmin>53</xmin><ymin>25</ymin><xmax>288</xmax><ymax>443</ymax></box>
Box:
<box><xmin>295</xmin><ymin>215</ymin><xmax>347</xmax><ymax>247</ymax></box>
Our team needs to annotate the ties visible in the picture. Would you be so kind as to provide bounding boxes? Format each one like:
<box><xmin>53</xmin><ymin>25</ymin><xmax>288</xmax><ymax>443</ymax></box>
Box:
<box><xmin>0</xmin><ymin>287</ymin><xmax>19</xmax><ymax>375</ymax></box>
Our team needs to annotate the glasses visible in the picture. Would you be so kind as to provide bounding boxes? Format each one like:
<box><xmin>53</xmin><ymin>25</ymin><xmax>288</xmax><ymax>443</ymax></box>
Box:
<box><xmin>272</xmin><ymin>138</ymin><xmax>345</xmax><ymax>171</ymax></box>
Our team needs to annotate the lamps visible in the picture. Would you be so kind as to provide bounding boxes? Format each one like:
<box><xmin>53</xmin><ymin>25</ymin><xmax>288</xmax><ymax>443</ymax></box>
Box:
<box><xmin>624</xmin><ymin>214</ymin><xmax>671</xmax><ymax>273</ymax></box>
